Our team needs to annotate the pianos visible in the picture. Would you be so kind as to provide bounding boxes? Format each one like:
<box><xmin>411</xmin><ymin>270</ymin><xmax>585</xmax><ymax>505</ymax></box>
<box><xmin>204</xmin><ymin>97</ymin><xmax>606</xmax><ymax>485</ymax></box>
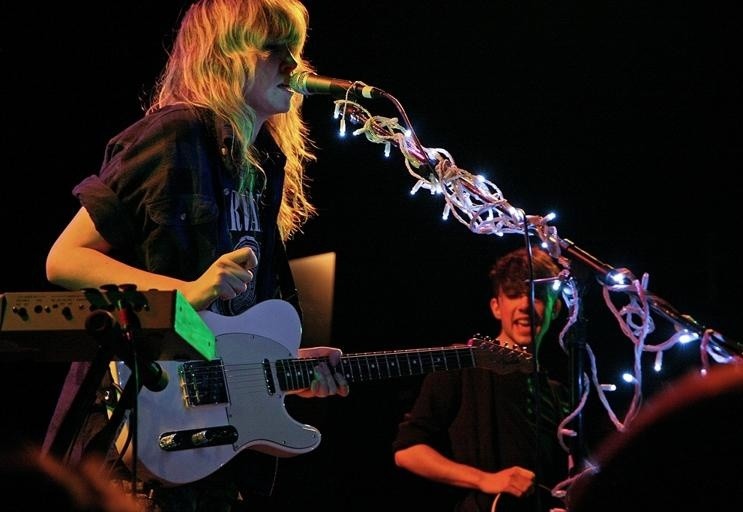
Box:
<box><xmin>3</xmin><ymin>290</ymin><xmax>215</xmax><ymax>364</ymax></box>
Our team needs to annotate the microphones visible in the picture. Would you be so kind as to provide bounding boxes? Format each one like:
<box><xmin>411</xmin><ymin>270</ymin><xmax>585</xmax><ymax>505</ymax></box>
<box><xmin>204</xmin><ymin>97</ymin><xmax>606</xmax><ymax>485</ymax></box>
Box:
<box><xmin>289</xmin><ymin>69</ymin><xmax>384</xmax><ymax>100</ymax></box>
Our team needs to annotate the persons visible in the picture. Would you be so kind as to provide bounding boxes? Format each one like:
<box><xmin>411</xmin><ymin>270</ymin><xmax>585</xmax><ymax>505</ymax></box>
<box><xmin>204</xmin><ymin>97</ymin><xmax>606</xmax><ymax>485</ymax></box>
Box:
<box><xmin>12</xmin><ymin>358</ymin><xmax>154</xmax><ymax>511</ymax></box>
<box><xmin>384</xmin><ymin>246</ymin><xmax>582</xmax><ymax>512</ymax></box>
<box><xmin>42</xmin><ymin>1</ymin><xmax>354</xmax><ymax>511</ymax></box>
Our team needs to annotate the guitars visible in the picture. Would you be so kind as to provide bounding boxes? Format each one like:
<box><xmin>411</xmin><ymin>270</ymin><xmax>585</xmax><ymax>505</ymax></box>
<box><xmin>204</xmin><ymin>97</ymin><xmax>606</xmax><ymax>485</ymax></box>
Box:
<box><xmin>105</xmin><ymin>300</ymin><xmax>535</xmax><ymax>487</ymax></box>
